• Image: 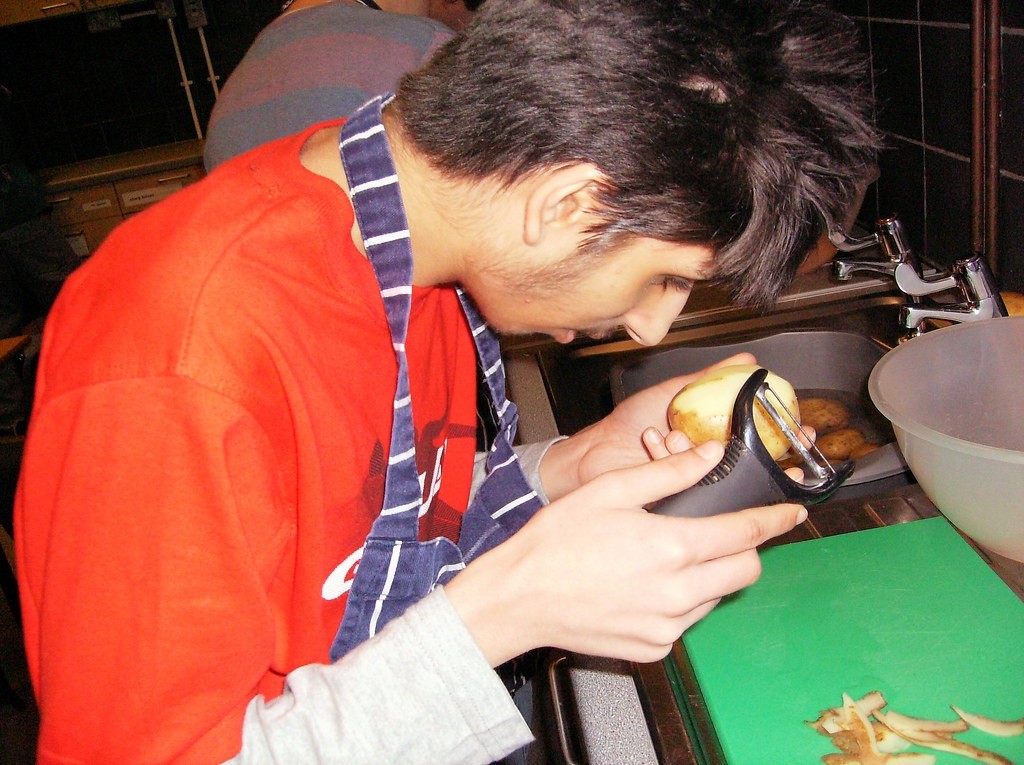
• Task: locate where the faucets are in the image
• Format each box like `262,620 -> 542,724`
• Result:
891,251 -> 1011,327
828,213 -> 927,345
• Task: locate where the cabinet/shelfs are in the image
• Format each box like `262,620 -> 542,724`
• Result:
47,163 -> 206,259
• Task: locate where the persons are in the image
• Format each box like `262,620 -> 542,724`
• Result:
201,0 -> 485,178
14,0 -> 875,765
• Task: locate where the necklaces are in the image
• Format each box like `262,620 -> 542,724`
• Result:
281,0 -> 368,11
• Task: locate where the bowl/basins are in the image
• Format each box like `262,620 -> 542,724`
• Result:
867,315 -> 1024,562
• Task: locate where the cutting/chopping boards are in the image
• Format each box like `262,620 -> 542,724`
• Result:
682,516 -> 1024,765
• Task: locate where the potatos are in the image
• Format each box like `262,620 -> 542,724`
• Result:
776,398 -> 886,471
667,365 -> 801,463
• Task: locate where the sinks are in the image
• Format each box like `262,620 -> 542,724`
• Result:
606,324 -> 896,469
